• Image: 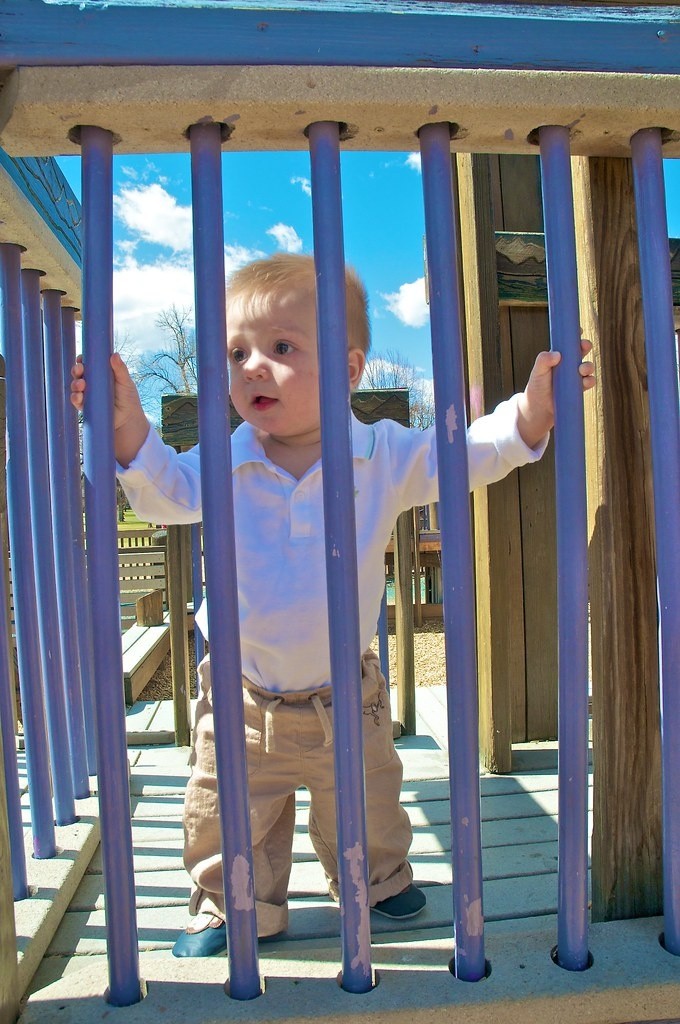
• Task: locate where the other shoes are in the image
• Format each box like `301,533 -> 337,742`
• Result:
171,913 -> 227,958
370,883 -> 427,919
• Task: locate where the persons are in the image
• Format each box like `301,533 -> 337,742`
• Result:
69,254 -> 598,962
